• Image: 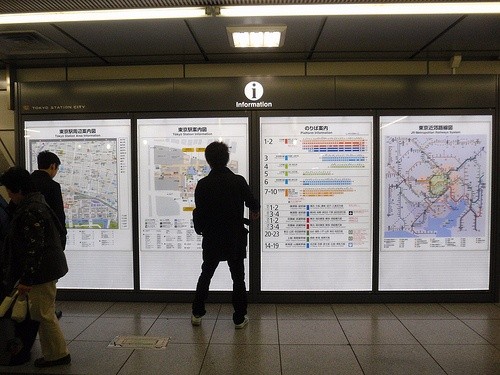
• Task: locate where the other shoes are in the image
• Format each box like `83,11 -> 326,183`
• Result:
33,354 -> 71,367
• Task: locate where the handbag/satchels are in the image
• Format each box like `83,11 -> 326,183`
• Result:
0,290 -> 40,366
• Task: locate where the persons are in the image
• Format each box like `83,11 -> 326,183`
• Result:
29,151 -> 68,318
192,141 -> 252,328
0,166 -> 71,367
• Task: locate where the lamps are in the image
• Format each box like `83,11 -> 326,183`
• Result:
0,6 -> 212,24
213,2 -> 500,17
226,26 -> 288,49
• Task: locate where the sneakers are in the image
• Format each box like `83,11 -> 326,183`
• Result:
235,316 -> 249,328
191,313 -> 202,325
